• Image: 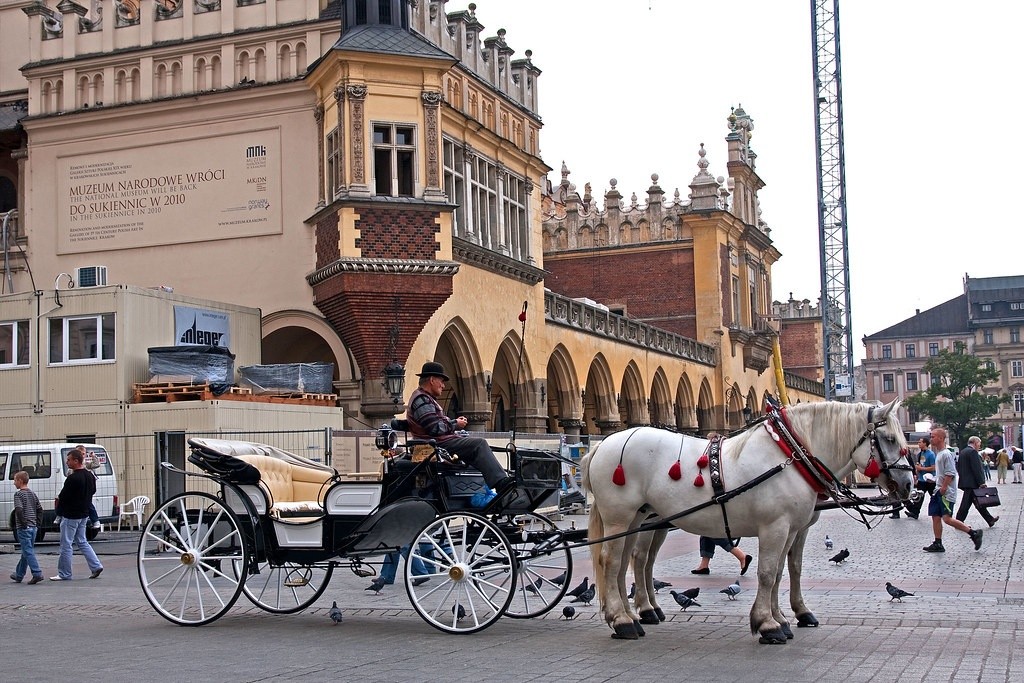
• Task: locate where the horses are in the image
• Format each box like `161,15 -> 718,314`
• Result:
577,395 -> 916,645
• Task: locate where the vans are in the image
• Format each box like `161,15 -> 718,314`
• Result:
0,442 -> 120,543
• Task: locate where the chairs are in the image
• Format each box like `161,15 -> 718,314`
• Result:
117,496 -> 151,531
391,418 -> 468,472
37,465 -> 51,477
22,466 -> 34,478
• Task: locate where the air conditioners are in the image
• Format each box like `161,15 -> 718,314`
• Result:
75,266 -> 108,289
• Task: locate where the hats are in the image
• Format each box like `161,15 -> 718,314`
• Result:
415,362 -> 450,382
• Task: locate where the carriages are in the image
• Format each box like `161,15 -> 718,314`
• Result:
135,394 -> 917,647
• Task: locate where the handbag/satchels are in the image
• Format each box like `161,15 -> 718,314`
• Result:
995,459 -> 1000,467
973,486 -> 1001,508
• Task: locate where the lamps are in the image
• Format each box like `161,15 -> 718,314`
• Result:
725,375 -> 752,425
379,298 -> 406,414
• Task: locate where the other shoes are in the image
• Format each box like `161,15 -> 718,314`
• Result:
969,529 -> 983,551
94,521 -> 101,529
412,577 -> 430,586
89,569 -> 103,580
372,578 -> 390,584
49,575 -> 62,581
904,511 -> 917,519
922,541 -> 945,552
740,554 -> 753,575
889,514 -> 900,519
989,516 -> 999,527
27,575 -> 43,585
496,476 -> 518,493
54,516 -> 62,524
440,553 -> 454,572
691,568 -> 710,575
10,574 -> 22,583
1018,481 -> 1022,483
1012,481 -> 1018,483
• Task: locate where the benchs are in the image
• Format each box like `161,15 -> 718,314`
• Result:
233,453 -> 332,523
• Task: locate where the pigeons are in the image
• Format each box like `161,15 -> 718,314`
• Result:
329,601 -> 344,625
842,548 -> 850,562
627,582 -> 636,599
452,599 -> 467,622
570,584 -> 596,606
719,579 -> 741,601
653,578 -> 672,594
519,575 -> 543,596
566,576 -> 590,597
828,548 -> 845,565
364,576 -> 386,596
548,570 -> 567,586
884,582 -> 915,603
824,534 -> 834,550
679,587 -> 701,601
563,606 -> 575,620
669,590 -> 702,612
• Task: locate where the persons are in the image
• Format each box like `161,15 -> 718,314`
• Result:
406,362 -> 515,492
889,436 -> 1024,520
49,445 -> 103,581
10,471 -> 44,585
371,443 -> 455,586
691,531 -> 753,577
923,428 -> 983,553
